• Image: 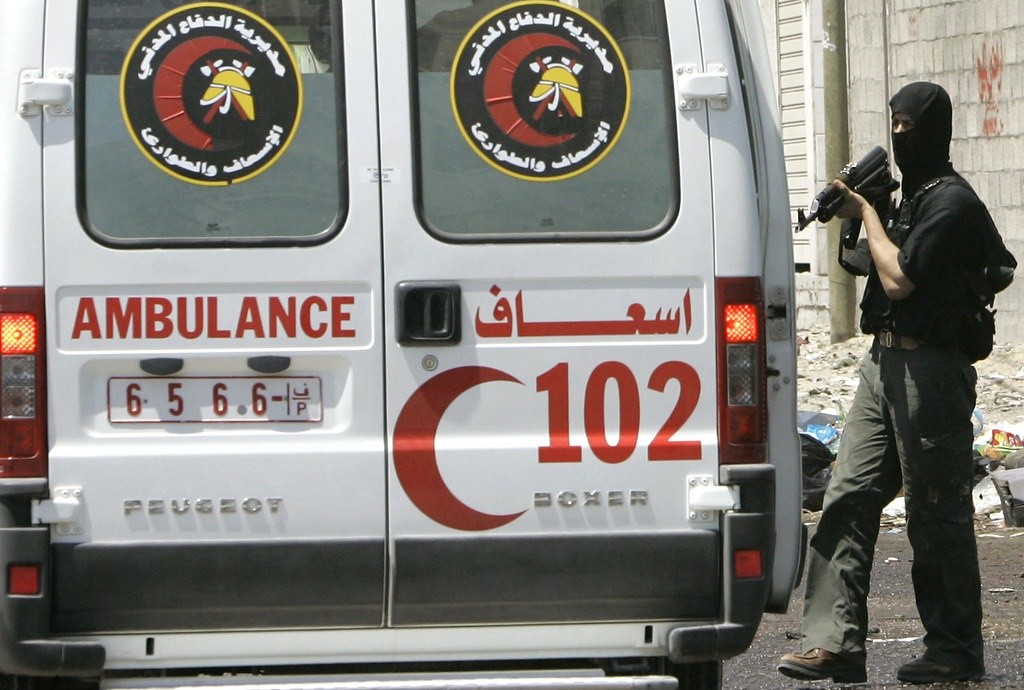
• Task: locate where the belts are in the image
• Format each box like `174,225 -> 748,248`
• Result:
876,328 -> 927,350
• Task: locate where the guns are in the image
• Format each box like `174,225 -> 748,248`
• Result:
790,143 -> 902,255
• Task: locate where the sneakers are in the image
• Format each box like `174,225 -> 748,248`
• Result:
898,656 -> 985,682
778,648 -> 867,683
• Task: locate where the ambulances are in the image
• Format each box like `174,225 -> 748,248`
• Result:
1,1 -> 810,690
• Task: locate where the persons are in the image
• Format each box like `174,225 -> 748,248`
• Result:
778,82 -> 1017,683
